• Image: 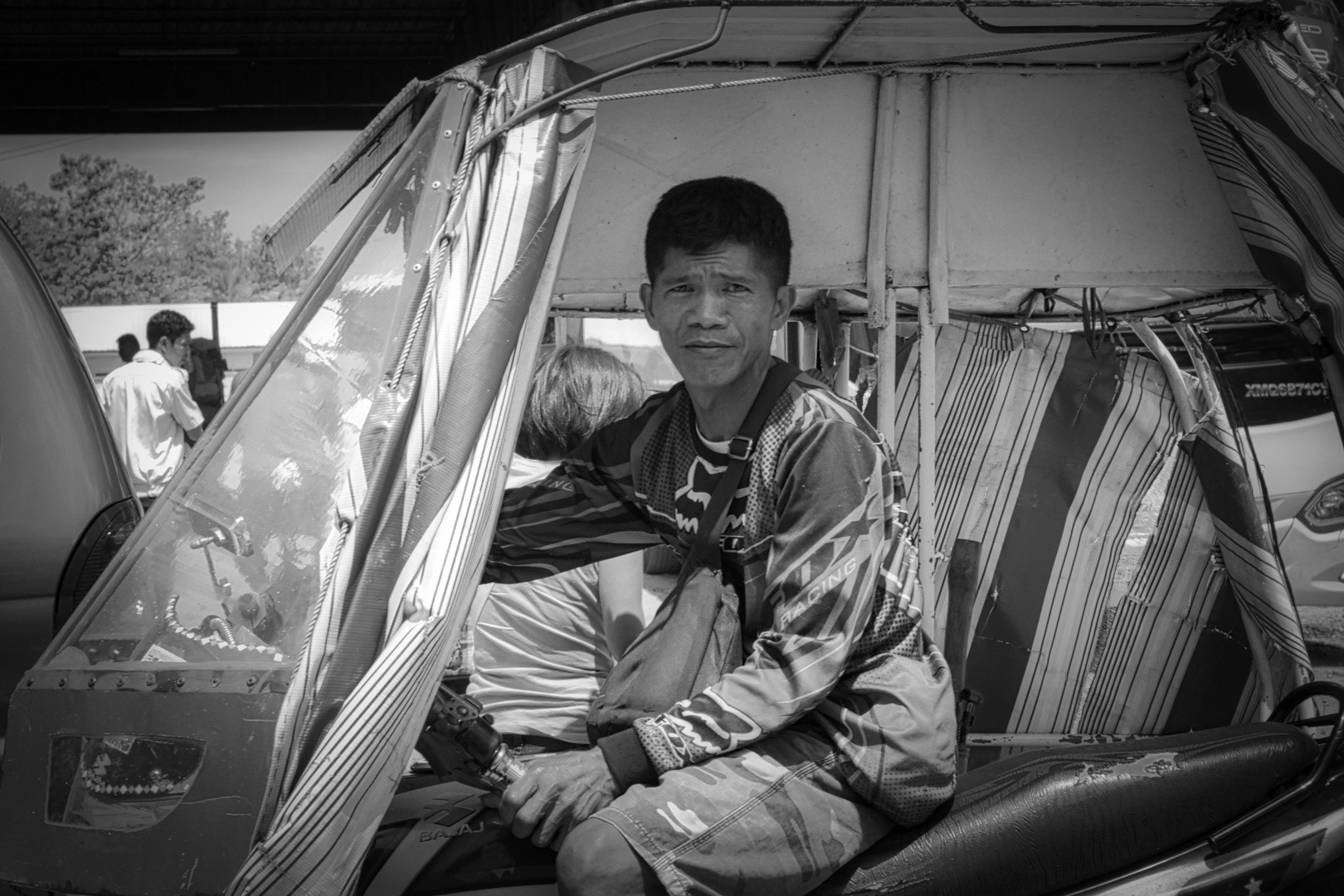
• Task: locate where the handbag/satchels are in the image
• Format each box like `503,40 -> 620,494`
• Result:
584,549 -> 746,748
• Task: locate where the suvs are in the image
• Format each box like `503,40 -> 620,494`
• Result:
1,218 -> 148,717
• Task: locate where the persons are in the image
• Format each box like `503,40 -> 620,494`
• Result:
460,346 -> 646,748
101,308 -> 227,615
231,335 -> 344,591
488,177 -> 957,896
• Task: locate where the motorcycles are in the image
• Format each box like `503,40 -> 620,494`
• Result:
0,0 -> 1344,896
